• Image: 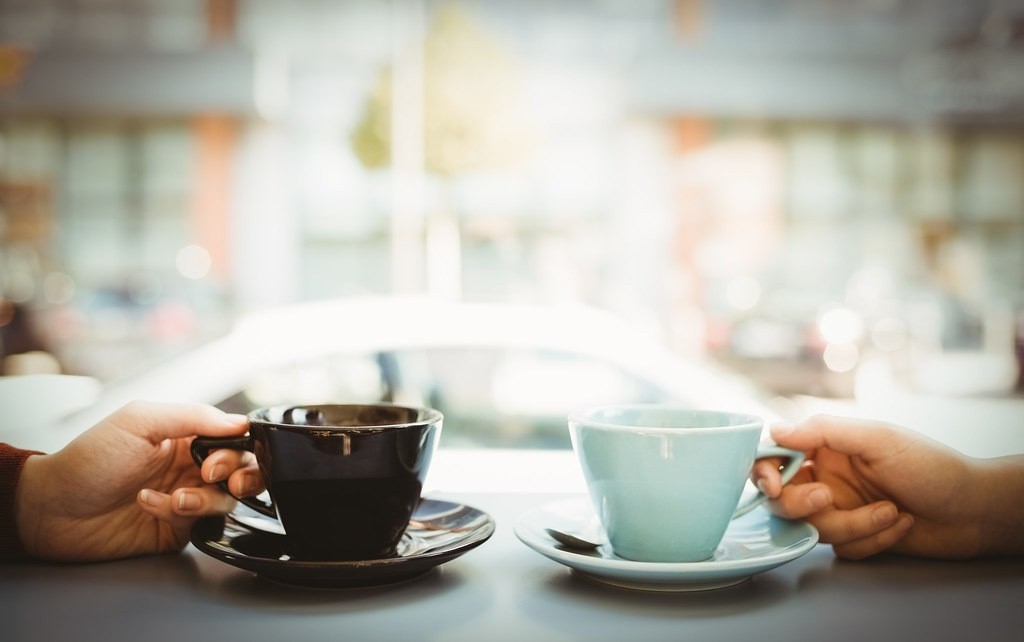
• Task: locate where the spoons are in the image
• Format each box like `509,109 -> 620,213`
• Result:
544,525 -> 605,551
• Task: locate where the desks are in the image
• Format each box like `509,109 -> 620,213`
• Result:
0,446 -> 1024,642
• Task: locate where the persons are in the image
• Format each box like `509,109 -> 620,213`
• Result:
749,413 -> 1024,562
0,398 -> 266,565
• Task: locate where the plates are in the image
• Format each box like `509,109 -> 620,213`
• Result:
513,486 -> 819,592
190,493 -> 495,588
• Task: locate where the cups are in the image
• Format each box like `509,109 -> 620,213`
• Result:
567,406 -> 805,562
190,402 -> 443,559
